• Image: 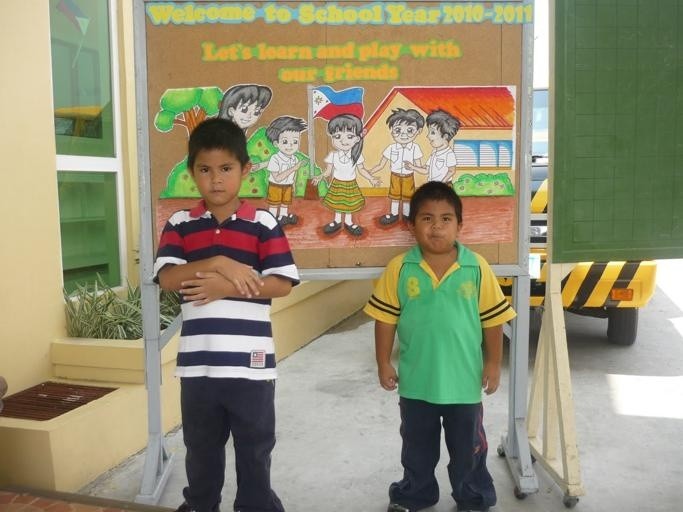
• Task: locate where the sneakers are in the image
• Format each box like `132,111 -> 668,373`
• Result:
275,213 -> 299,226
387,501 -> 415,512
378,214 -> 410,226
174,501 -> 244,512
323,220 -> 364,237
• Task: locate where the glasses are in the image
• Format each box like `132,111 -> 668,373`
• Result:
391,133 -> 415,138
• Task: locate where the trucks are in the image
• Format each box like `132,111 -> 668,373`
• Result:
490,1 -> 682,344
56,98 -> 104,139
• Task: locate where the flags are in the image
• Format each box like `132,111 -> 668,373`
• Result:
313,85 -> 364,124
56,0 -> 90,35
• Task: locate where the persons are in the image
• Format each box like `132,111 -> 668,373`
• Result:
153,118 -> 300,511
363,181 -> 518,512
312,114 -> 379,235
403,108 -> 460,190
217,84 -> 272,134
370,108 -> 424,225
265,115 -> 310,229
